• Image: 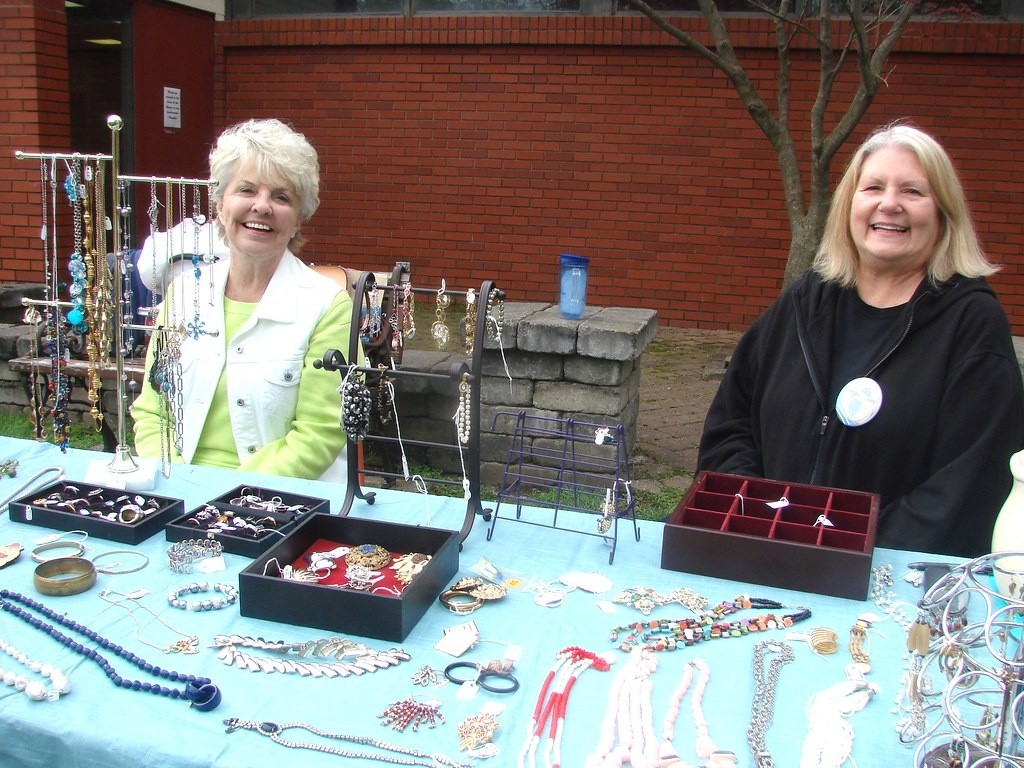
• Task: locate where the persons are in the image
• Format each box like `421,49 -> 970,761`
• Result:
696,126 -> 1024,561
128,119 -> 366,484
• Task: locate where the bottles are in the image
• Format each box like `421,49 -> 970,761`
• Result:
990,449 -> 1024,617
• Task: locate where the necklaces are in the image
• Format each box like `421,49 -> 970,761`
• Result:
209,634 -> 412,678
26,153 -> 213,482
523,592 -> 811,768
223,717 -> 475,768
0,589 -> 222,710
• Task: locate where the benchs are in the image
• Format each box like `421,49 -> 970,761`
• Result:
9,354 -> 148,452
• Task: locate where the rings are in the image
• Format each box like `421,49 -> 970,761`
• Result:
189,486 -> 310,537
34,485 -> 159,523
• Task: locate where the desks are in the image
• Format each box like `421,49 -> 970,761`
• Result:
0,434 -> 1024,768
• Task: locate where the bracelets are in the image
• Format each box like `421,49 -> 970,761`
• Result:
32,541 -> 97,596
339,365 -> 471,445
91,549 -> 148,574
372,281 -> 504,351
167,582 -> 238,612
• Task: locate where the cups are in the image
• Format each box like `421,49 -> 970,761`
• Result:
558,253 -> 590,320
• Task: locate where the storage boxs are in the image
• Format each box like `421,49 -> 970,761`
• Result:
662,470 -> 882,601
237,514 -> 459,645
166,484 -> 331,561
10,478 -> 184,547
